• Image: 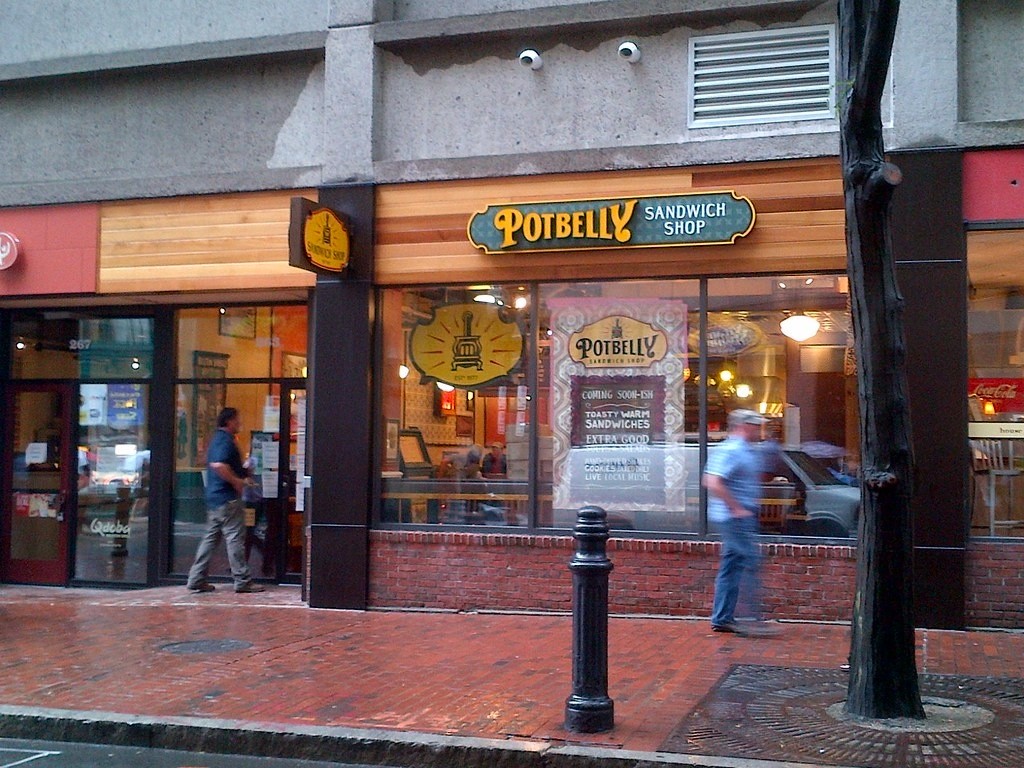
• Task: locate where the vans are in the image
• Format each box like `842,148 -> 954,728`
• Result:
552,442 -> 862,538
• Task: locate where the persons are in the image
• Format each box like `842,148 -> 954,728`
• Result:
700,408 -> 782,636
437,442 -> 507,476
187,407 -> 284,592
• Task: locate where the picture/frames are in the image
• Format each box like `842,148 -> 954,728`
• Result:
220,306 -> 257,340
386,417 -> 400,463
456,415 -> 473,437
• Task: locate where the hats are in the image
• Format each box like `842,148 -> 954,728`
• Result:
729,409 -> 773,425
492,442 -> 504,449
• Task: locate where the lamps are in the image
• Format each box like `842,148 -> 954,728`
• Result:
780,310 -> 820,341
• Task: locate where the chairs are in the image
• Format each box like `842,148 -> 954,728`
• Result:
967,394 -> 1024,537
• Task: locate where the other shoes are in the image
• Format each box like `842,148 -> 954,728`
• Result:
711,618 -> 747,633
737,623 -> 783,637
237,582 -> 265,592
188,582 -> 215,591
